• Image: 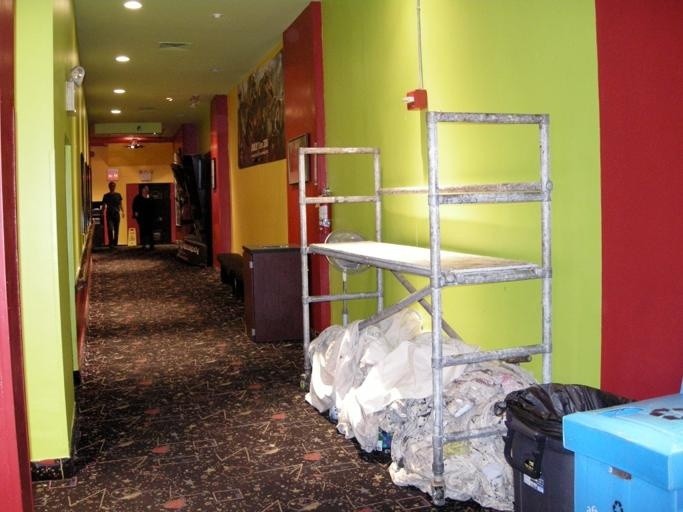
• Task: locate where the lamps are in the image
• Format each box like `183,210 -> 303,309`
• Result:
65,66 -> 86,112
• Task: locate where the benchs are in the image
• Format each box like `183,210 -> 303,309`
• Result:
217,253 -> 244,298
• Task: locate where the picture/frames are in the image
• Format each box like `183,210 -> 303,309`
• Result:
287,133 -> 310,185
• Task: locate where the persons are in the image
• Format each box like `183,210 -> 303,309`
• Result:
102,181 -> 124,246
132,185 -> 154,250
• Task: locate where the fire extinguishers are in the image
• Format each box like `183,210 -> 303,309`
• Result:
317,190 -> 331,242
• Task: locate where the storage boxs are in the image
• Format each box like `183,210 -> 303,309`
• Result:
561,390 -> 682,511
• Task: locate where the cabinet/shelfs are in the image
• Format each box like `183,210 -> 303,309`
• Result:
242,244 -> 311,344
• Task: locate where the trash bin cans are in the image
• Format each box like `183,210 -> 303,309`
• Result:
504,383 -> 634,512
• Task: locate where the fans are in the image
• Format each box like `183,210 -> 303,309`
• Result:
324,229 -> 371,332
